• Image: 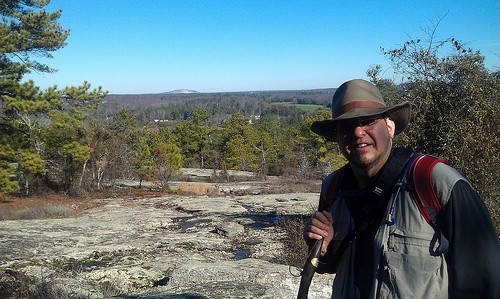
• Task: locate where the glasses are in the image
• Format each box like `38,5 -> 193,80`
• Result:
325,116 -> 388,141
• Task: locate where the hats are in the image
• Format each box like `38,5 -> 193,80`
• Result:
310,79 -> 411,142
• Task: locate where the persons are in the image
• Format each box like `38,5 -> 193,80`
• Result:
303,79 -> 500,299
